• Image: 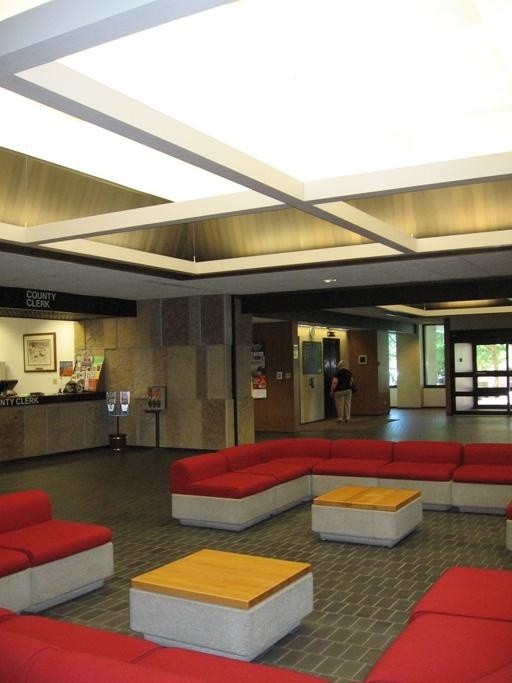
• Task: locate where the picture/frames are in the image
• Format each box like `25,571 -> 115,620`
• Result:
23,333 -> 56,372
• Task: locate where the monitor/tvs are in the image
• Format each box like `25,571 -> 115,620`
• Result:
0,379 -> 19,398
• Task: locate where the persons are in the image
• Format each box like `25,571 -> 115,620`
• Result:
331,359 -> 354,423
253,369 -> 267,389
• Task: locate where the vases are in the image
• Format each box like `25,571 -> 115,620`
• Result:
109,433 -> 126,458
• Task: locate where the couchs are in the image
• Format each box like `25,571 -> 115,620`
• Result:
170,438 -> 512,552
0,489 -> 512,683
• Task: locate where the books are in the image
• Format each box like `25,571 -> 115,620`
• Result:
71,356 -> 102,392
30,392 -> 45,396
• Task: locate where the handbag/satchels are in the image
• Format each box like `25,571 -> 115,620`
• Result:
351,383 -> 357,393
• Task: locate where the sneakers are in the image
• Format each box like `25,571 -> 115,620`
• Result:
336,420 -> 342,423
344,420 -> 350,423
